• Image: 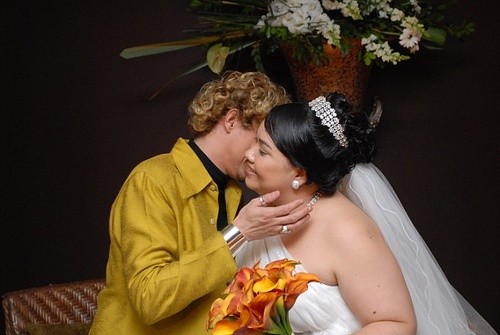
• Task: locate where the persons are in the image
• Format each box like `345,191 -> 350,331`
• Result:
236,90 -> 499,335
87,70 -> 313,335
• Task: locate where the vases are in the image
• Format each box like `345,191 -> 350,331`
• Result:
281,38 -> 371,113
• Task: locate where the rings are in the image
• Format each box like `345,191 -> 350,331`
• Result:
259,196 -> 267,206
280,225 -> 291,234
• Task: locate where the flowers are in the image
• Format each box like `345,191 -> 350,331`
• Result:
206,258 -> 322,335
120,0 -> 475,101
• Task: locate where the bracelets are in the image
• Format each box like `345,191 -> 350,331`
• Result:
221,223 -> 249,259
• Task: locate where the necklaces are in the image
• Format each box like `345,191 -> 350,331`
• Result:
308,190 -> 322,206
